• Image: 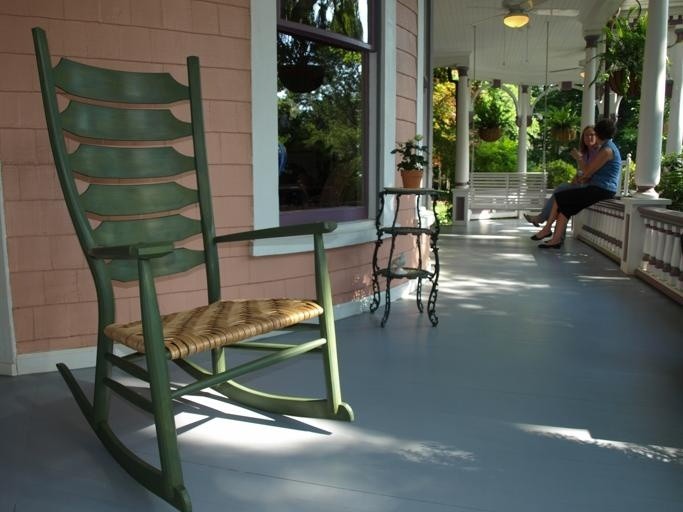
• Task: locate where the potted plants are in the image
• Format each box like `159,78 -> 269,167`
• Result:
586,1 -> 649,99
391,134 -> 432,188
540,100 -> 580,145
471,87 -> 512,142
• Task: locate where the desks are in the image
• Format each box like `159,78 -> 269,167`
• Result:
369,188 -> 441,326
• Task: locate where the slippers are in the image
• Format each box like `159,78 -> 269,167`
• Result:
524,213 -> 540,227
543,237 -> 564,244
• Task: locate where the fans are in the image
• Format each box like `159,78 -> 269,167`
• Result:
466,0 -> 579,30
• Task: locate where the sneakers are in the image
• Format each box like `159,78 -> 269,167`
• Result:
530,230 -> 552,240
538,244 -> 561,249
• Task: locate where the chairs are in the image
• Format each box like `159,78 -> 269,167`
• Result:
32,26 -> 354,511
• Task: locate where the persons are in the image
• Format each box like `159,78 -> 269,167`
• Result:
530,118 -> 623,249
523,123 -> 603,244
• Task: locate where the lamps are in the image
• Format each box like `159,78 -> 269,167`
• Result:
502,14 -> 529,30
